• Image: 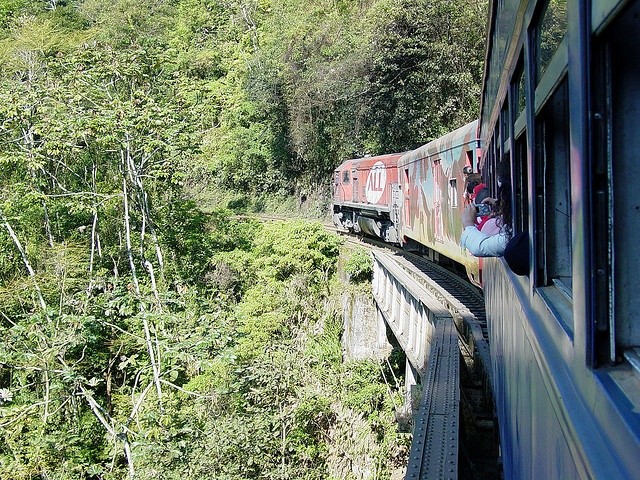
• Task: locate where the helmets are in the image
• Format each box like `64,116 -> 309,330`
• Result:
463,166 -> 472,174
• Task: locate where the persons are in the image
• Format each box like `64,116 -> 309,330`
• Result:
460,165 -> 511,257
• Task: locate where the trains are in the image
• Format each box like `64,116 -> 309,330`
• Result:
334,0 -> 639,480
